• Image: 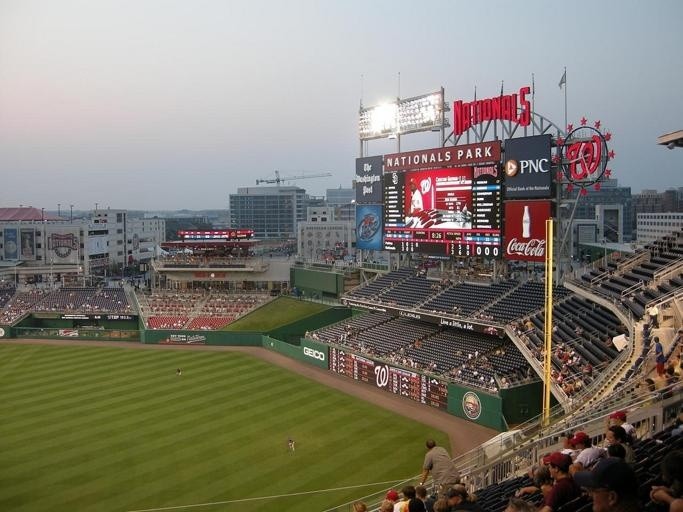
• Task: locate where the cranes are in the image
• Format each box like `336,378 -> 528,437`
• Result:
256,171 -> 332,186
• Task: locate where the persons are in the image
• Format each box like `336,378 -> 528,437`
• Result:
0,289 -> 132,323
347,410 -> 683,512
406,176 -> 424,212
509,318 -> 593,401
648,303 -> 659,327
638,337 -> 683,398
393,278 -> 496,322
306,325 -> 505,389
145,319 -> 212,330
289,439 -> 295,451
176,367 -> 181,375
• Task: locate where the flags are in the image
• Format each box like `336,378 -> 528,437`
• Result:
559,70 -> 568,88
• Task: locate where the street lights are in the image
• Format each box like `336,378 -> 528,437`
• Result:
359,85 -> 443,156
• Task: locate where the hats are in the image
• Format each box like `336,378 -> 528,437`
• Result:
544,452 -> 566,466
574,457 -> 636,491
609,411 -> 627,421
384,490 -> 398,502
570,432 -> 587,445
